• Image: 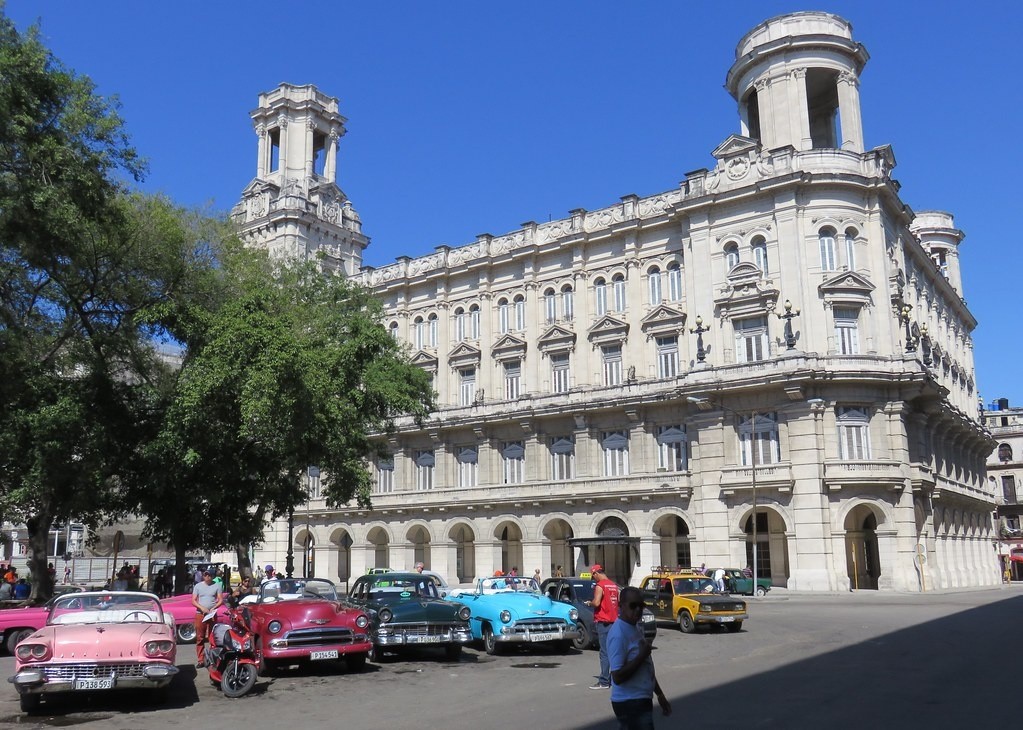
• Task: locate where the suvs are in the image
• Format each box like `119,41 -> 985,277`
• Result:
637,565 -> 749,634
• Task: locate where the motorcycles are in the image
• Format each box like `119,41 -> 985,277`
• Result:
201,595 -> 262,698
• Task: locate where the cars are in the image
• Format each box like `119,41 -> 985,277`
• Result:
237,577 -> 372,676
343,568 -> 473,663
0,583 -> 232,713
702,567 -> 772,597
442,576 -> 658,655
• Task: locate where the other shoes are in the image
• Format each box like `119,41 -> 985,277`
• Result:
196,663 -> 204,668
589,683 -> 610,690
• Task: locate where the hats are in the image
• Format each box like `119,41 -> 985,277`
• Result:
202,570 -> 210,575
589,565 -> 604,581
264,566 -> 273,572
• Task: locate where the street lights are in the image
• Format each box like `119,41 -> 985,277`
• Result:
686,395 -> 824,597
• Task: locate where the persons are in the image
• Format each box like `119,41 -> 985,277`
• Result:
606,587 -> 671,730
104,554 -> 289,604
742,564 -> 753,578
699,562 -> 707,576
46,561 -> 56,575
191,569 -> 223,668
533,568 -> 541,585
60,562 -> 73,585
657,563 -> 682,588
404,561 -> 424,595
715,567 -> 725,592
0,563 -> 31,602
505,565 -> 518,591
584,564 -> 620,691
493,570 -> 507,589
554,565 -> 566,577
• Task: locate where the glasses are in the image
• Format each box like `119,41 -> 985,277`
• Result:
624,602 -> 647,610
244,580 -> 249,582
